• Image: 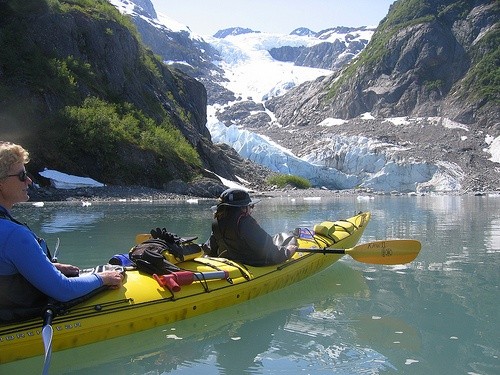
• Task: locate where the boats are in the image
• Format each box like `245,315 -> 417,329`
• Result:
0,212 -> 375,363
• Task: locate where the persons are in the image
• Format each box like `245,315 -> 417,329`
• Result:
0,142 -> 124,324
211,187 -> 299,267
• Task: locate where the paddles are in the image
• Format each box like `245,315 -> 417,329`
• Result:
36,237 -> 60,375
295,239 -> 422,266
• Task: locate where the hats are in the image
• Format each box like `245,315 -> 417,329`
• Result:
218,188 -> 264,207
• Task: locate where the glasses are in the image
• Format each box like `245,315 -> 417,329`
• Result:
1,168 -> 27,182
246,203 -> 255,209
